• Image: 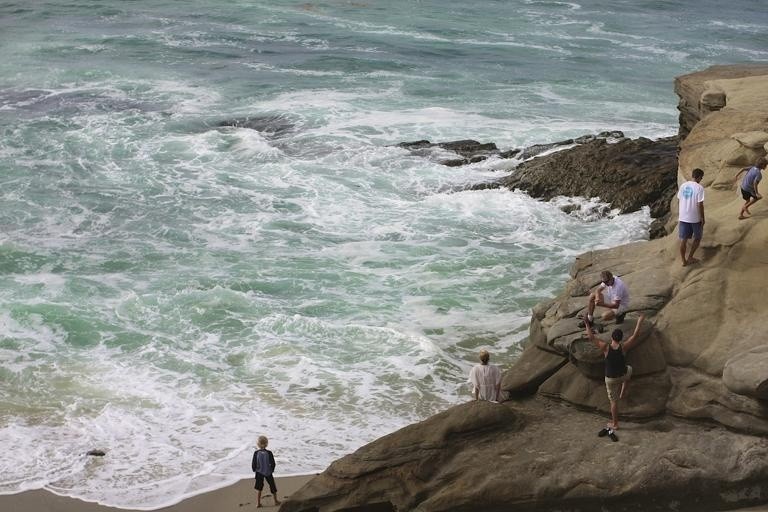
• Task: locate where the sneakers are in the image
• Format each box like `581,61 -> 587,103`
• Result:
577,318 -> 594,328
598,427 -> 618,442
615,312 -> 626,324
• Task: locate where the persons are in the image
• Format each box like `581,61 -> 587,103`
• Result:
677,168 -> 706,266
471,349 -> 510,403
578,270 -> 629,327
252,435 -> 282,507
737,156 -> 767,219
583,312 -> 645,430
